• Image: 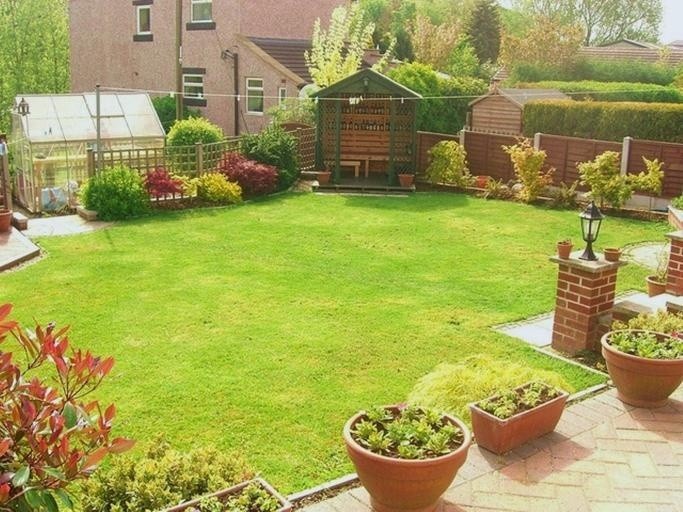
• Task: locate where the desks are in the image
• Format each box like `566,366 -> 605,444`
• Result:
323,152 -> 412,178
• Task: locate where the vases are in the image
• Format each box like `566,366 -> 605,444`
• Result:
604,247 -> 621,262
398,173 -> 415,186
315,171 -> 332,185
0,208 -> 12,233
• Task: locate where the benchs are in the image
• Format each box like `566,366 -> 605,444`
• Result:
323,160 -> 361,177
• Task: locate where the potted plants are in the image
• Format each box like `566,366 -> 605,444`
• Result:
163,476 -> 293,512
343,380 -> 571,511
557,239 -> 573,259
600,329 -> 683,407
645,237 -> 672,298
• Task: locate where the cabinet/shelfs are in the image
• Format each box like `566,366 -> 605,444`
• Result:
321,102 -> 413,135
